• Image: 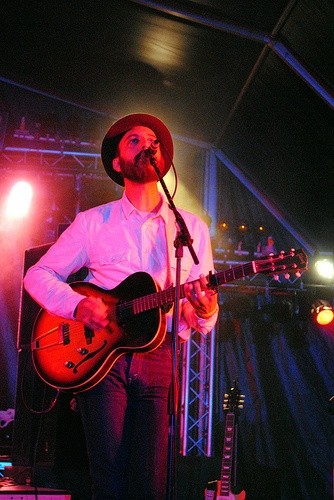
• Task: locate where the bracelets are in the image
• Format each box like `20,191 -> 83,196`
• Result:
196,306 -> 218,318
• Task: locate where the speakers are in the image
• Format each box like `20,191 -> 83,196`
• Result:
9,243 -> 89,492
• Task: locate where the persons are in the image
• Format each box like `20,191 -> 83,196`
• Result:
262,234 -> 279,254
23,112 -> 222,499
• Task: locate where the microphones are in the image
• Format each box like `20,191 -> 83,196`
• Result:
144,137 -> 160,158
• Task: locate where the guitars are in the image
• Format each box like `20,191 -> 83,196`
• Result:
29,244 -> 308,394
204,376 -> 246,500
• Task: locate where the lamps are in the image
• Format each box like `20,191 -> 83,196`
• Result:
308,299 -> 333,325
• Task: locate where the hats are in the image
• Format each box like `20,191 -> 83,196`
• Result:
100,113 -> 174,187
